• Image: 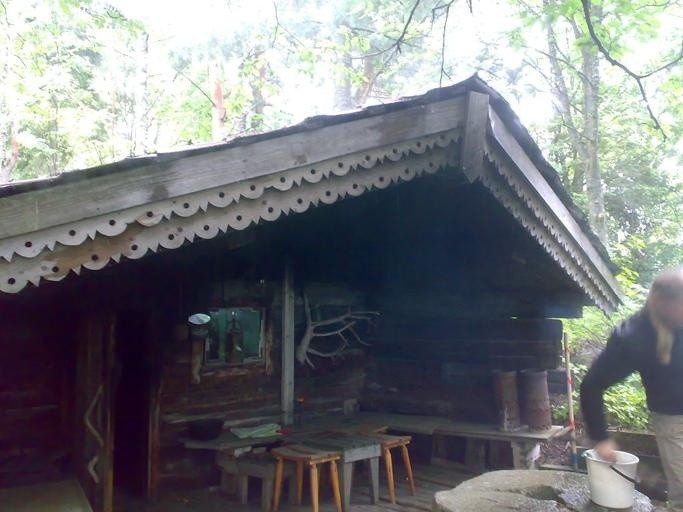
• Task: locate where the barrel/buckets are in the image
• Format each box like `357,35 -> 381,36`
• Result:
489,365 -> 519,430
583,450 -> 641,509
520,367 -> 551,433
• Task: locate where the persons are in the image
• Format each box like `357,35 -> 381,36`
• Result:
579,270 -> 683,512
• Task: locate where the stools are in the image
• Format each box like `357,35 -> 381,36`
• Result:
183,424 -> 414,510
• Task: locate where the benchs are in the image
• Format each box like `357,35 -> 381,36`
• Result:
359,412 -> 564,470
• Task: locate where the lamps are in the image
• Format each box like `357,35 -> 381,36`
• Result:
185,293 -> 212,326
